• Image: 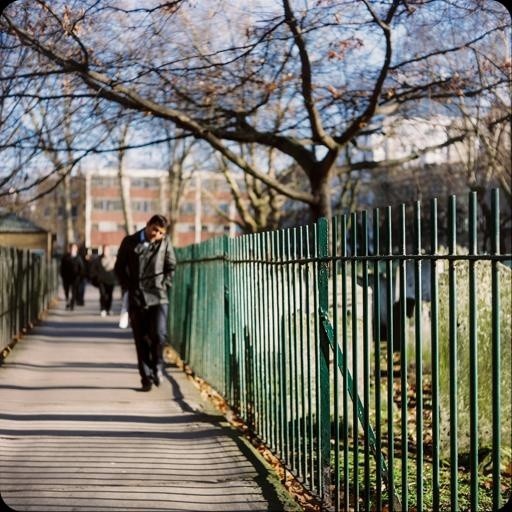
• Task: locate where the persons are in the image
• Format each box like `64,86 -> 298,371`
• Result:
118,290 -> 130,329
75,245 -> 93,307
91,244 -> 117,315
59,243 -> 85,309
114,214 -> 179,390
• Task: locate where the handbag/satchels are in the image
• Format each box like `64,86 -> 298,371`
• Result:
119,291 -> 129,328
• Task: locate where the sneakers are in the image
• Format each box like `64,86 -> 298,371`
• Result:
101,309 -> 114,317
143,384 -> 152,391
154,372 -> 161,388
